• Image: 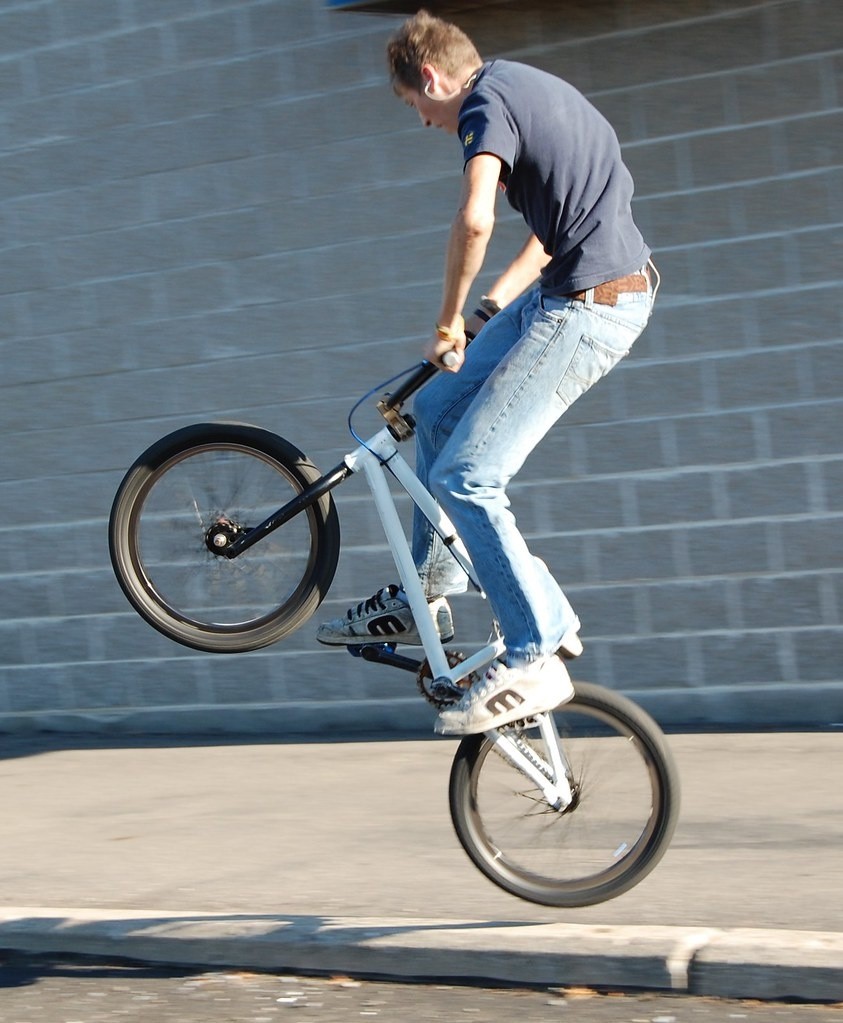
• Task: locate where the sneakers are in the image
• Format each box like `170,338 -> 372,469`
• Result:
434,654 -> 575,736
316,583 -> 454,646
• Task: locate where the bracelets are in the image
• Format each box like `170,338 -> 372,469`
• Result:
436,313 -> 464,341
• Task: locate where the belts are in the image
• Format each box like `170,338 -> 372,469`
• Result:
561,266 -> 650,306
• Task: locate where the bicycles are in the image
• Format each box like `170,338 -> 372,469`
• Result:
106,328 -> 684,910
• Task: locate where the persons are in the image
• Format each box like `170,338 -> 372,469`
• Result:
314,11 -> 662,732
